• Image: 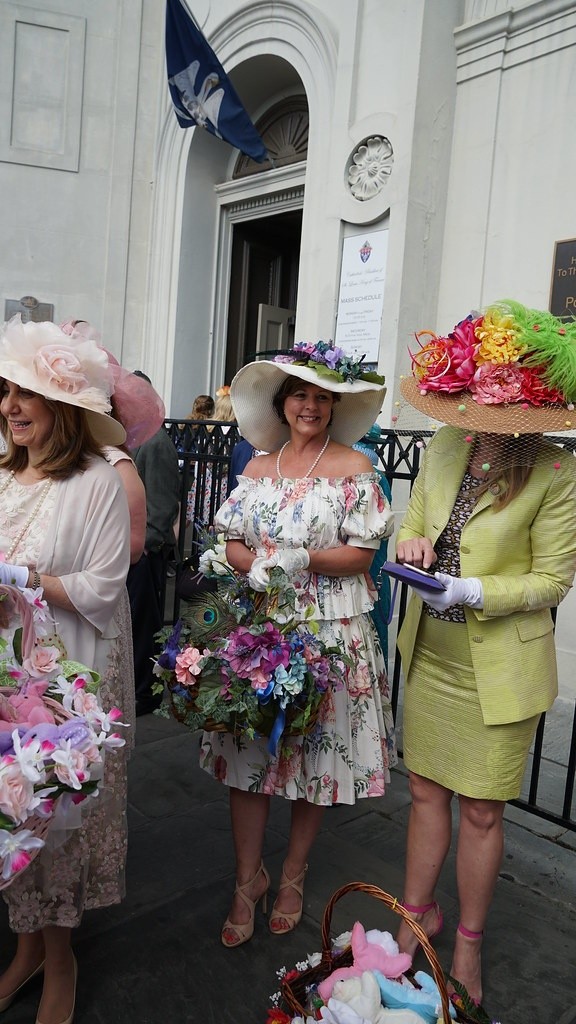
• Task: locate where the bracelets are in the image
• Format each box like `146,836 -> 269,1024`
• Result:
33,572 -> 40,591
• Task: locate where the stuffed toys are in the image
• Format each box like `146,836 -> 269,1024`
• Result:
289,920 -> 457,1024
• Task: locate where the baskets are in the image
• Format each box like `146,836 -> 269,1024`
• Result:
170,591 -> 329,744
282,882 -> 481,1024
0,584 -> 80,892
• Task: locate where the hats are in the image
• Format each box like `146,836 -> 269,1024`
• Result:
60,316 -> 165,447
229,340 -> 388,454
0,312 -> 126,447
397,300 -> 576,469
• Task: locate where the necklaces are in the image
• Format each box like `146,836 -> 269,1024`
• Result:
0,468 -> 53,564
277,434 -> 330,478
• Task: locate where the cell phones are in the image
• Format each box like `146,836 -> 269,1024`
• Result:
403,562 -> 436,579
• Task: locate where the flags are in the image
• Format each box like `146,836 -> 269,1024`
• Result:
165,0 -> 267,165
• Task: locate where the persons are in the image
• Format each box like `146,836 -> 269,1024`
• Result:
397,298 -> 576,1011
186,385 -> 242,524
130,370 -> 179,716
232,440 -> 270,492
214,340 -> 397,948
351,423 -> 390,674
0,313 -> 130,1024
165,395 -> 215,576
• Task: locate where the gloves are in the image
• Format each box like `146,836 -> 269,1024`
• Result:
261,546 -> 310,580
248,558 -> 271,592
411,571 -> 483,613
0,561 -> 28,588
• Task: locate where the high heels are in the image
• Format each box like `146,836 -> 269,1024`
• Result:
446,923 -> 484,1008
0,954 -> 47,1010
396,899 -> 442,965
269,862 -> 309,934
36,946 -> 77,1024
222,859 -> 270,947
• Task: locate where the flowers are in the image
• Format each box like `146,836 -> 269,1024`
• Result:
0,312 -> 116,413
269,338 -> 386,385
0,577 -> 132,882
216,386 -> 231,398
407,307 -> 567,407
265,929 -> 353,1024
149,515 -> 355,759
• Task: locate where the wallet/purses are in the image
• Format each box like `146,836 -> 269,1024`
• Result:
381,562 -> 447,594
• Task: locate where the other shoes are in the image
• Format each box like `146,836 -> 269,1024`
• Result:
166,566 -> 176,578
